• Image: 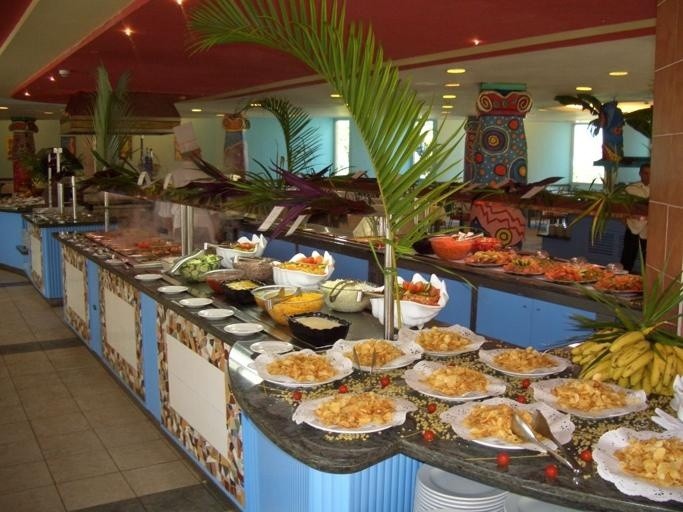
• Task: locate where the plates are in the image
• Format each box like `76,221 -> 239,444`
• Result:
334,338 -> 425,371
133,273 -> 162,282
157,286 -> 190,294
403,359 -> 506,400
292,391 -> 419,435
253,347 -> 354,386
397,326 -> 485,356
598,429 -> 683,501
534,379 -> 644,418
477,345 -> 573,378
464,230 -> 643,298
58,228 -> 125,265
446,400 -> 567,452
408,462 -> 510,511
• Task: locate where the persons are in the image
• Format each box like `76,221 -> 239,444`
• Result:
157,143 -> 217,241
616,162 -> 651,272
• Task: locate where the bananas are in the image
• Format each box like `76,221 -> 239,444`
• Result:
571,329 -> 682,395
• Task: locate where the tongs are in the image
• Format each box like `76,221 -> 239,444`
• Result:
505,407 -> 584,476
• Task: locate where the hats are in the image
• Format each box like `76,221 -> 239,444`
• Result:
169,120 -> 201,155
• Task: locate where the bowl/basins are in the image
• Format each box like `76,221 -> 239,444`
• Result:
410,235 -> 474,260
174,242 -> 442,348
